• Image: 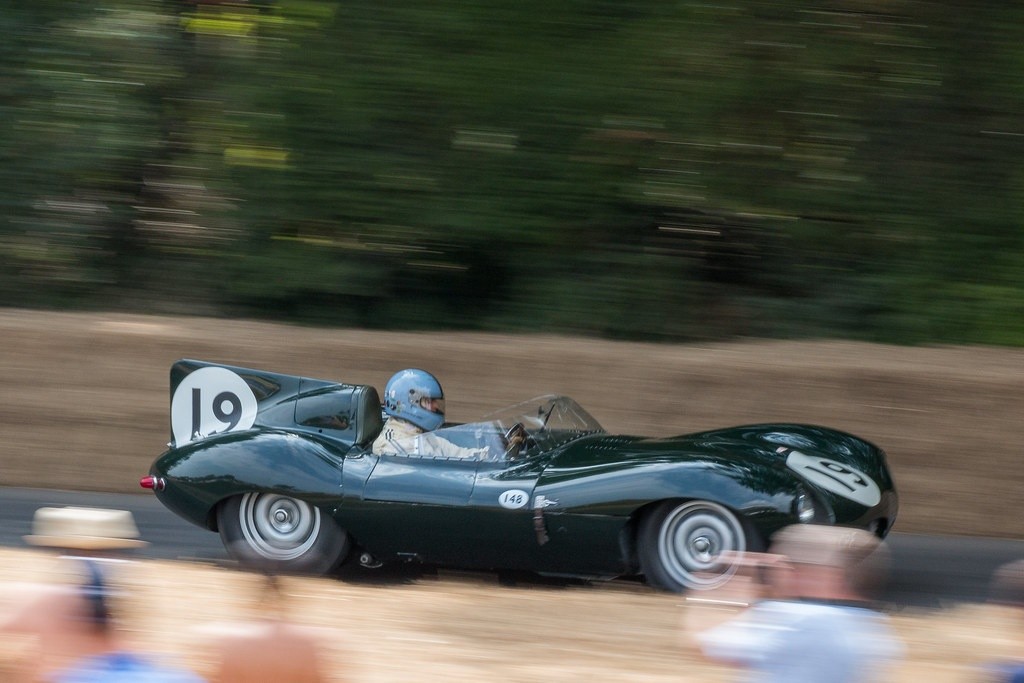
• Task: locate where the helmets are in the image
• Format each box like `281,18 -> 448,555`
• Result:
384,368 -> 446,431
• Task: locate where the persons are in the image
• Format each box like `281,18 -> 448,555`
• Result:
0,522 -> 1024,683
373,369 -> 523,461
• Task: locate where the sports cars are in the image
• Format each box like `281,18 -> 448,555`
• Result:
137,358 -> 901,601
